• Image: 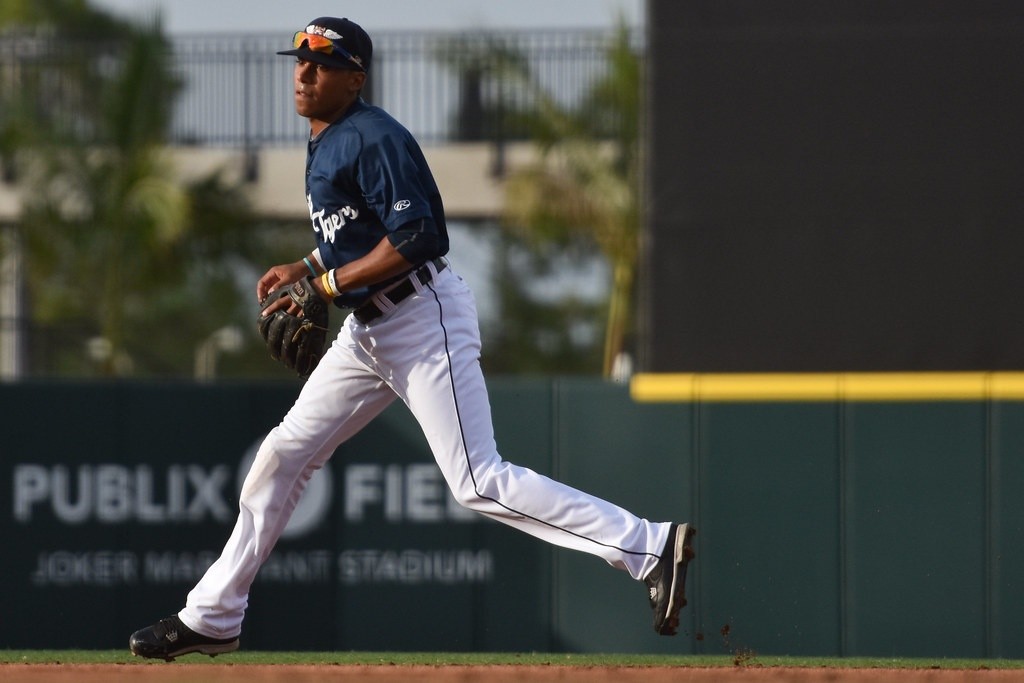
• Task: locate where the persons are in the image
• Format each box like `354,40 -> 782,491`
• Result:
129,17 -> 697,660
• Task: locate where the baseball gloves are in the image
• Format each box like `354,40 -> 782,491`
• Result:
257,275 -> 329,379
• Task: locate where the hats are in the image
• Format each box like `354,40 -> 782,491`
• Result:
276,17 -> 373,74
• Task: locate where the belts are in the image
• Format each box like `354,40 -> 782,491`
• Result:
351,257 -> 447,325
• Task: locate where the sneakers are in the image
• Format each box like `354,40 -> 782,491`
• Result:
129,614 -> 240,662
644,522 -> 696,635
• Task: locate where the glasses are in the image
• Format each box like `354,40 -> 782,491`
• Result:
292,30 -> 367,74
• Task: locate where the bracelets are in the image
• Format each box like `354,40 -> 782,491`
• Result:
303,257 -> 318,278
322,268 -> 342,298
